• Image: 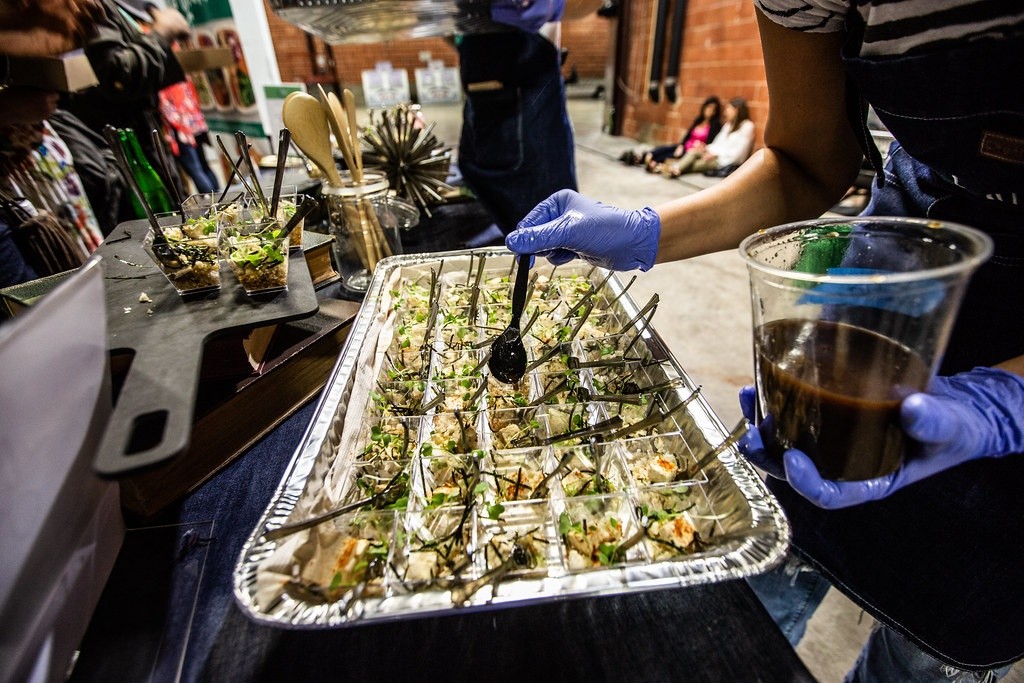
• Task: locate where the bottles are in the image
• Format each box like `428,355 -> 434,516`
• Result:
319,167 -> 404,294
116,127 -> 174,218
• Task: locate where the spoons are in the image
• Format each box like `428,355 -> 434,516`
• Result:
115,153 -> 182,270
487,253 -> 530,384
266,484 -> 408,539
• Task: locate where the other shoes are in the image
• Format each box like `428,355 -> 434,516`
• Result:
644,153 -> 656,172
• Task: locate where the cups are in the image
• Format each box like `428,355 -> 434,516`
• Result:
742,214 -> 996,480
286,263 -> 724,602
244,186 -> 304,251
180,191 -> 243,239
143,215 -> 222,298
221,214 -> 289,297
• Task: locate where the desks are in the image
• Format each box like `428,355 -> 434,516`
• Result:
68,154 -> 822,683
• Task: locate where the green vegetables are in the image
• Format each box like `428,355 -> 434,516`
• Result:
330,269 -> 692,586
165,188 -> 304,269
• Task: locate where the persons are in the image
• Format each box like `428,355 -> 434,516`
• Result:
621,96 -> 722,167
645,98 -> 755,177
0,0 -> 220,286
506,0 -> 1024,683
427,0 -> 564,245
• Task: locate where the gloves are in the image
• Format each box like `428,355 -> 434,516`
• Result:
739,367 -> 1024,511
505,189 -> 660,273
491,0 -> 565,33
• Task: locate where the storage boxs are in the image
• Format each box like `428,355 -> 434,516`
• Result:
0,255 -> 125,683
